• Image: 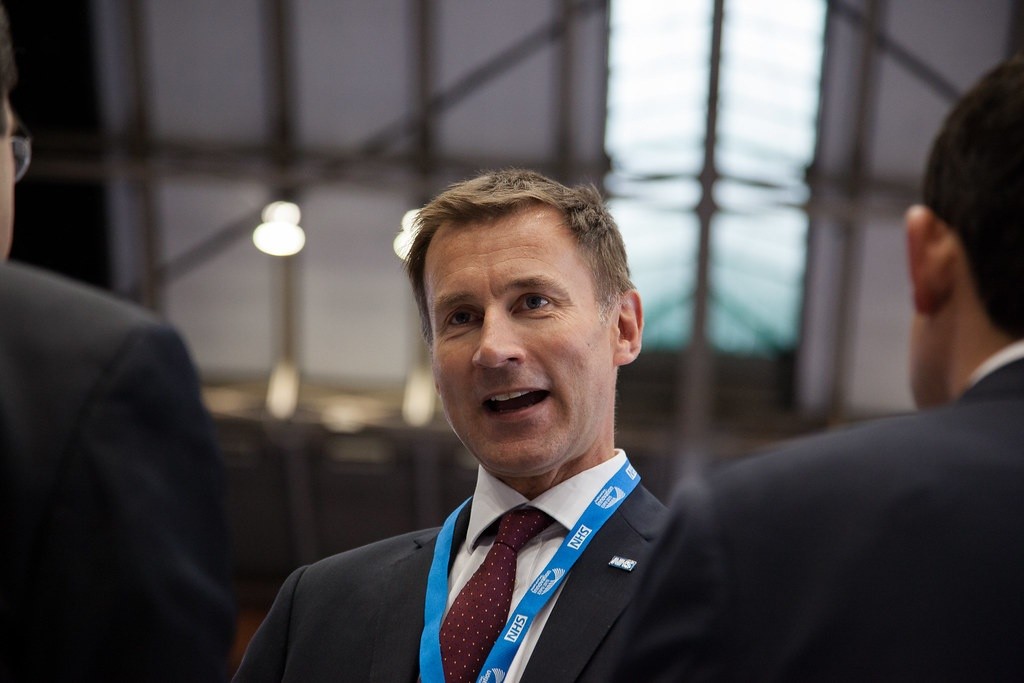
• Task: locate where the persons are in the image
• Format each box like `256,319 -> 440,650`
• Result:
235,169 -> 667,683
0,0 -> 236,683
615,52 -> 1024,683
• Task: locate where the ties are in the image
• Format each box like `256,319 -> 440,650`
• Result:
418,508 -> 557,682
2,136 -> 31,182
